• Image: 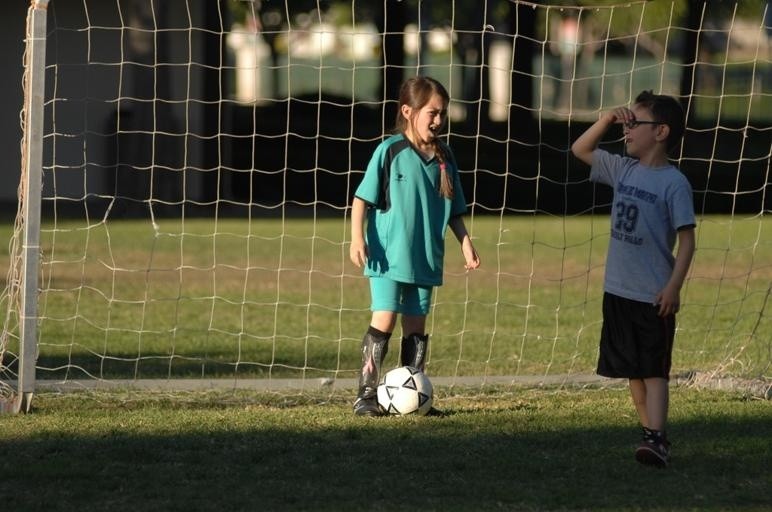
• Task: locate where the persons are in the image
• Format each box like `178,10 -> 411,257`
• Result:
570,93 -> 696,465
349,78 -> 482,414
224,3 -> 772,105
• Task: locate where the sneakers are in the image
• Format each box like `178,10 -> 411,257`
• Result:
353,386 -> 381,416
634,427 -> 672,468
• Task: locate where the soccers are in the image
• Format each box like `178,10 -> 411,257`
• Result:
376,365 -> 434,417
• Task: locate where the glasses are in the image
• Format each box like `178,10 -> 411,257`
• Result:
628,118 -> 664,128
